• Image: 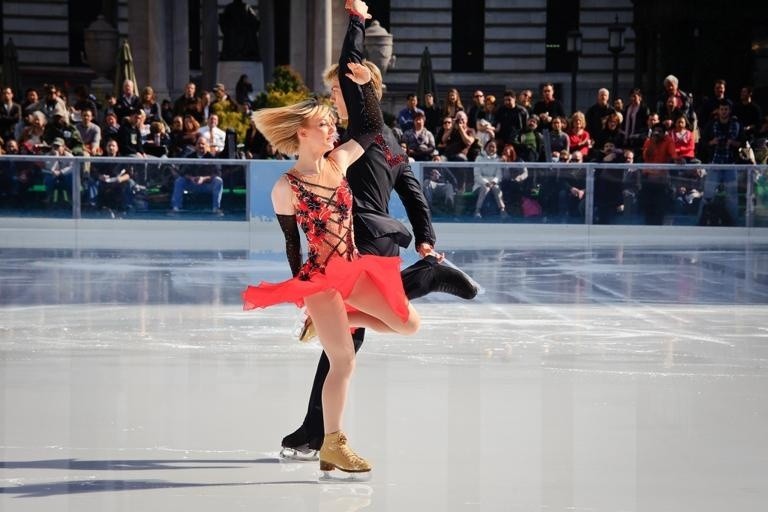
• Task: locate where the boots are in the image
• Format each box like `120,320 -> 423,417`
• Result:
298,314 -> 318,341
319,431 -> 372,474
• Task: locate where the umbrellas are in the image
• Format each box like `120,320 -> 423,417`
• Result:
415,46 -> 440,108
113,38 -> 139,98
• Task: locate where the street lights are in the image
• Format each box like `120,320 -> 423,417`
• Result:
565,22 -> 585,117
606,13 -> 627,111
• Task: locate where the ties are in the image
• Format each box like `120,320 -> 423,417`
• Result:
209,128 -> 214,145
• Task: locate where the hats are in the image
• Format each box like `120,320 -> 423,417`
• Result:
52,110 -> 65,117
52,137 -> 66,146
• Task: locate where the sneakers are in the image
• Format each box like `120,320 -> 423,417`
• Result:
281,423 -> 324,452
165,209 -> 225,218
424,254 -> 478,298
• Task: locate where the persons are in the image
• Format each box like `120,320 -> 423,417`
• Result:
281,0 -> 477,451
242,61 -> 419,473
1,73 -> 765,224
